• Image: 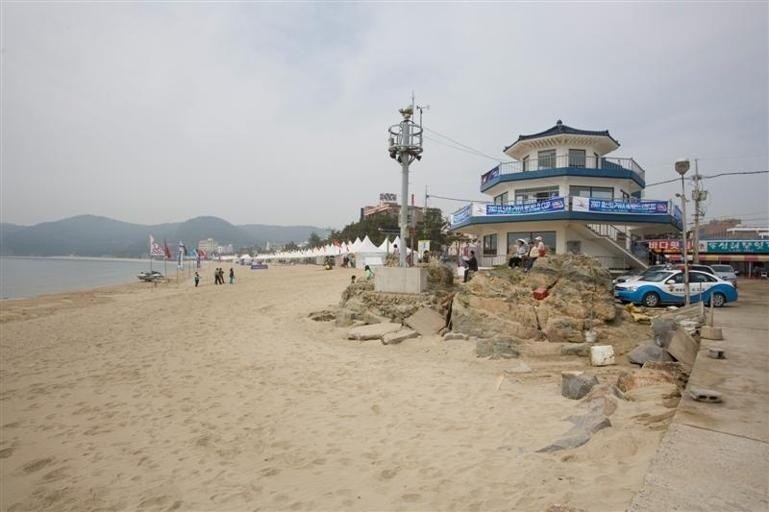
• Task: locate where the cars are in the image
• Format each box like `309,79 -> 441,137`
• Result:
611,260 -> 739,308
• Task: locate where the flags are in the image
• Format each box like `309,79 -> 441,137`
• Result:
147,235 -> 208,271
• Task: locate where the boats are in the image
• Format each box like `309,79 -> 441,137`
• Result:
136,270 -> 164,281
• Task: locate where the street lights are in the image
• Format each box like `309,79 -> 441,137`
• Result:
674,157 -> 689,310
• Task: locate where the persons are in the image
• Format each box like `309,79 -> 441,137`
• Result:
323,253 -> 356,270
364,265 -> 375,281
351,275 -> 356,284
392,244 -> 399,257
214,268 -> 224,284
229,268 -> 235,284
193,272 -> 199,287
508,236 -> 545,270
422,250 -> 430,262
462,250 -> 478,283
